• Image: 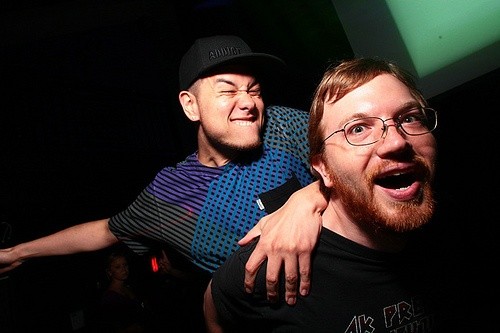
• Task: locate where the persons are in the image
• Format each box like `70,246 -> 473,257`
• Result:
94,249 -> 151,332
199,57 -> 500,333
1,39 -> 333,309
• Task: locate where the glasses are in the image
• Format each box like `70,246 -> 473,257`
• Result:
315,107 -> 438,152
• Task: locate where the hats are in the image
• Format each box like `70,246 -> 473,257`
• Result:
178,36 -> 288,95
